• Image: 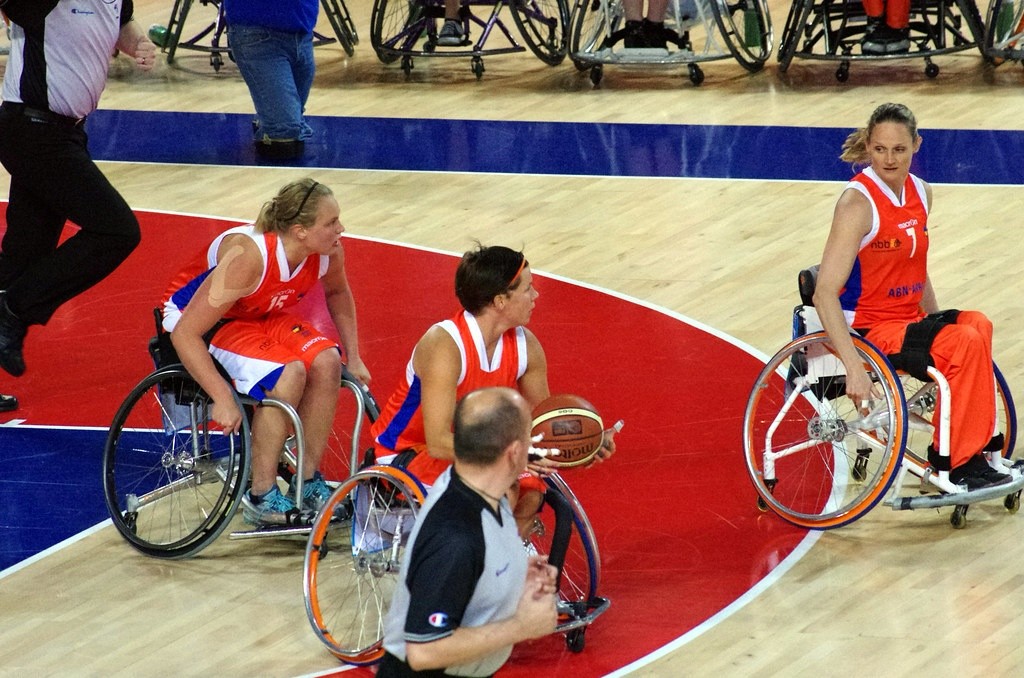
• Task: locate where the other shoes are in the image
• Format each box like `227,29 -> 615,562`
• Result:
557,595 -> 608,626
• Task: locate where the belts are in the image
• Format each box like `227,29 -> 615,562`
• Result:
1,101 -> 86,130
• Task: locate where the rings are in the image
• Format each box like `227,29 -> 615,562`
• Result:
142,58 -> 145,64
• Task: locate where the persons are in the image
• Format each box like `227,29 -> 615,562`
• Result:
371,246 -> 625,558
860,0 -> 911,55
813,103 -> 1013,494
624,0 -> 669,48
222,0 -> 320,144
162,176 -> 370,530
0,0 -> 158,411
376,386 -> 558,678
436,0 -> 463,45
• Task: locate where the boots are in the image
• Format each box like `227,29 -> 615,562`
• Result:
862,15 -> 886,54
624,18 -> 669,56
884,24 -> 911,54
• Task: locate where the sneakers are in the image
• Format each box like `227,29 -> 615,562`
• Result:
284,471 -> 350,521
958,467 -> 1013,492
437,19 -> 463,45
243,484 -> 312,527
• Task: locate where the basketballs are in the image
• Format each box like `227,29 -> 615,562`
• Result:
530,393 -> 605,469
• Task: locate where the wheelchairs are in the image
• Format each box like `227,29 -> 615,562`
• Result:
982,0 -> 1024,86
102,308 -> 382,561
370,0 -> 570,79
300,469 -> 611,666
777,0 -> 986,86
741,265 -> 1024,530
566,0 -> 773,88
149,0 -> 359,74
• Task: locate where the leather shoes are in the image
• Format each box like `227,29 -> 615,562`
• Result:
0,394 -> 18,412
0,291 -> 25,376
